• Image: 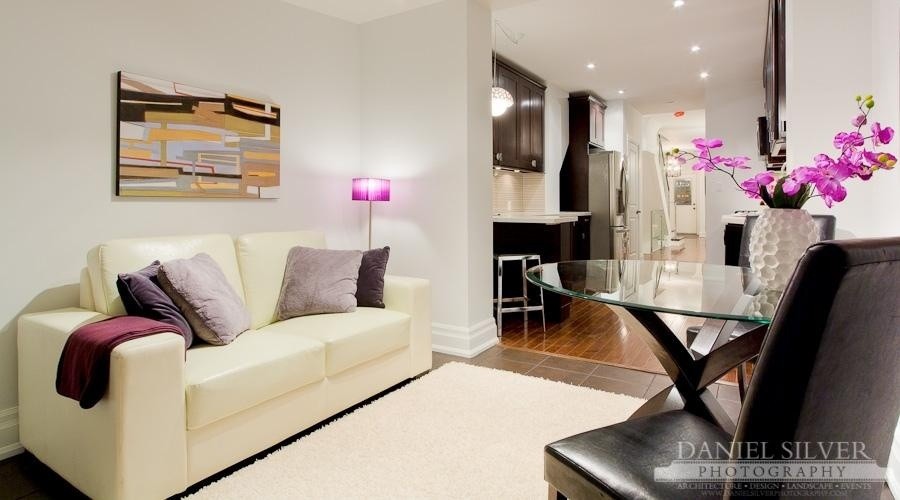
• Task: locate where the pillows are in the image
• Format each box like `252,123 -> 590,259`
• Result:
273,243 -> 390,321
114,253 -> 251,346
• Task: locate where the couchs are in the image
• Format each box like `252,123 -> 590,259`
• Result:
15,227 -> 432,500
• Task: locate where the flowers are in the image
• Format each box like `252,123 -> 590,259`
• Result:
659,94 -> 897,213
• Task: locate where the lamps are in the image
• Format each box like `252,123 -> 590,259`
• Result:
490,21 -> 514,118
351,177 -> 391,249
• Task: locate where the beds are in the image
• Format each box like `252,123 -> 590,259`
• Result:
527,259 -> 783,442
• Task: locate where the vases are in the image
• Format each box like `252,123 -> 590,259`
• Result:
748,207 -> 820,294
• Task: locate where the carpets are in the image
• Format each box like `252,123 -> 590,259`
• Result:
186,360 -> 648,500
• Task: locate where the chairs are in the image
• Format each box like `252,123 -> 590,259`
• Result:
544,238 -> 900,500
687,214 -> 835,399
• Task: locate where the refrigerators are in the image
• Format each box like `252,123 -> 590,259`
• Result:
586,150 -> 628,293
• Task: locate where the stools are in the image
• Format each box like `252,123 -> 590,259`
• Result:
493,250 -> 546,340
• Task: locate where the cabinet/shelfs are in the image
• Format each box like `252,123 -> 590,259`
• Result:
493,61 -> 519,167
517,81 -> 544,170
569,95 -> 606,150
